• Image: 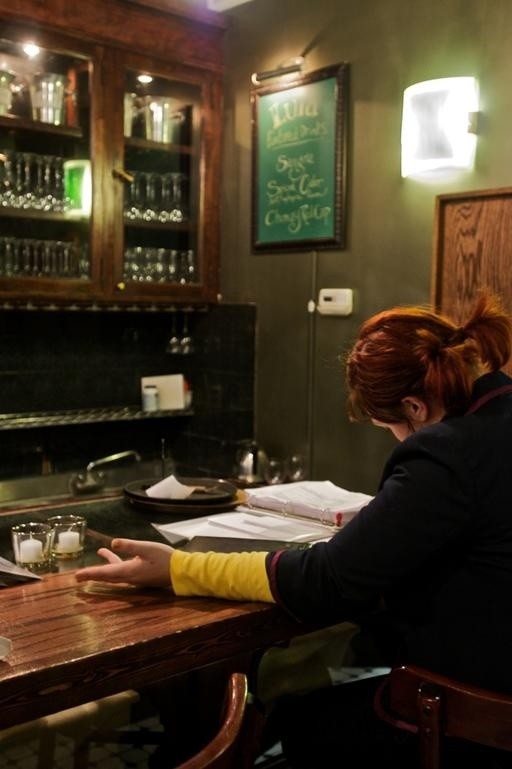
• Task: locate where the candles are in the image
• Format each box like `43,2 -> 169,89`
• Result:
56,526 -> 84,552
18,530 -> 47,563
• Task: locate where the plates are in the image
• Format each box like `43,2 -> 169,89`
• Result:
122,476 -> 238,505
126,486 -> 249,514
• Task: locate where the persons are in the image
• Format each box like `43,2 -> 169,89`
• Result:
69,286 -> 511,769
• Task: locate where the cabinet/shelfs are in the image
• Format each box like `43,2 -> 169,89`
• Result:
0,14 -> 225,308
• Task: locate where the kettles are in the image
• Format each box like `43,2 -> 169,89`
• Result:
236,438 -> 267,484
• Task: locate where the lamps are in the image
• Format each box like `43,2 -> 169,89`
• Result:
401,76 -> 483,185
249,55 -> 304,89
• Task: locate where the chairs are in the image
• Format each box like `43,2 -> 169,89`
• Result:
172,673 -> 248,767
358,662 -> 509,768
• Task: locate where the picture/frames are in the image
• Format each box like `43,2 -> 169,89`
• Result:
249,63 -> 352,256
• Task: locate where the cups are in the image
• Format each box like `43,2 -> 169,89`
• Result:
10,522 -> 54,569
0,236 -> 91,279
123,246 -> 199,286
125,172 -> 186,218
46,514 -> 88,561
0,147 -> 71,212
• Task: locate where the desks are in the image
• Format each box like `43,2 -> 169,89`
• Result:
1,562 -> 350,729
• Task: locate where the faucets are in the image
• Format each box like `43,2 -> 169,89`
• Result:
70,449 -> 143,491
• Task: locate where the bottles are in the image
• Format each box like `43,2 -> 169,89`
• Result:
141,384 -> 160,413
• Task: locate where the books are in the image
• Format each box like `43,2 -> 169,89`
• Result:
240,480 -> 376,528
